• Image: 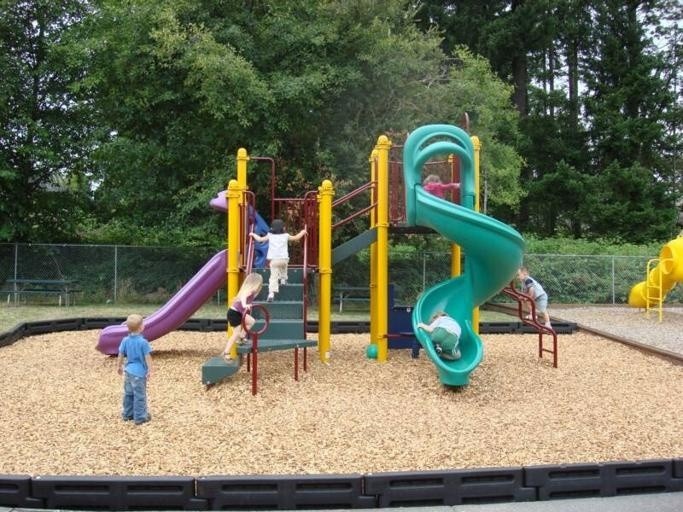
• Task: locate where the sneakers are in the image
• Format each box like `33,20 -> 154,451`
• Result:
266,293 -> 275,301
123,414 -> 150,425
280,280 -> 287,286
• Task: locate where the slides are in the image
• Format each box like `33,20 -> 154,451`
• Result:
95,248 -> 228,356
412,184 -> 525,387
628,238 -> 682,306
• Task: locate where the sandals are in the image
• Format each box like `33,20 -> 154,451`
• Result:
221,351 -> 234,364
237,337 -> 250,345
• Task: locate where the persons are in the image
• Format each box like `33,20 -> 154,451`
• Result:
418,311 -> 461,360
517,266 -> 552,331
249,220 -> 308,302
116,314 -> 152,424
422,175 -> 461,200
220,273 -> 264,364
